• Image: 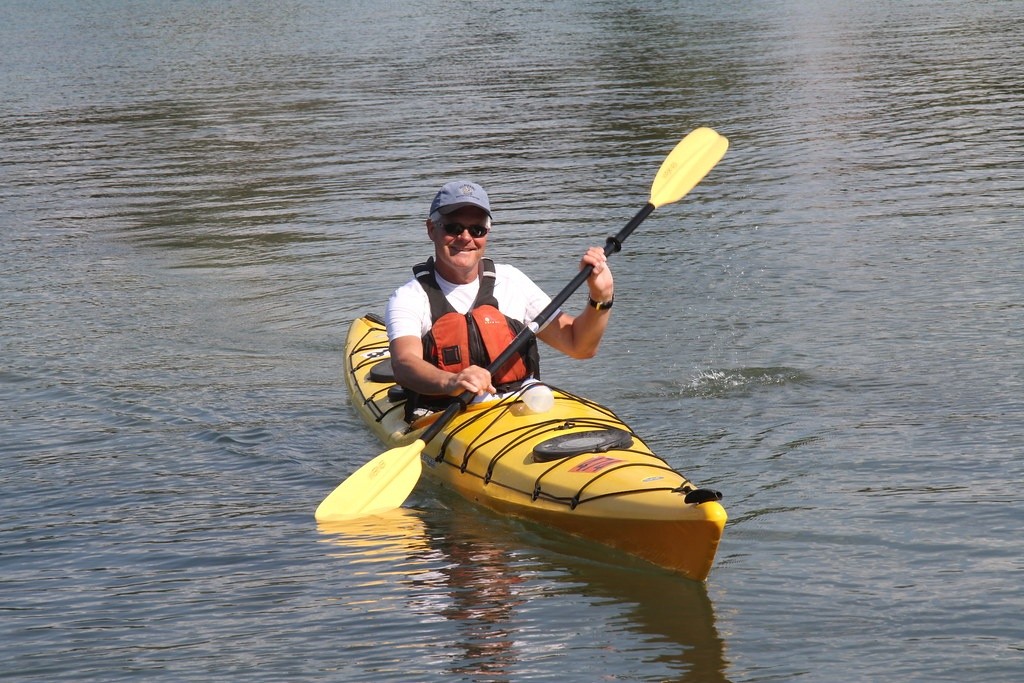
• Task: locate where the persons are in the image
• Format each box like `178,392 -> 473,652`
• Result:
384,181 -> 613,421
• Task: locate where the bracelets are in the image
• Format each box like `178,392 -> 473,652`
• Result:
587,294 -> 614,311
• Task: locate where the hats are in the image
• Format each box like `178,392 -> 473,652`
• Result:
430,180 -> 492,219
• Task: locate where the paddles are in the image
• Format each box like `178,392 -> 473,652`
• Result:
309,122 -> 731,526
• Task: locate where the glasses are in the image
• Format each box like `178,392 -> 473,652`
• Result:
432,221 -> 490,238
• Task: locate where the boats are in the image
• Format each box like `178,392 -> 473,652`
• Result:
346,313 -> 727,579
